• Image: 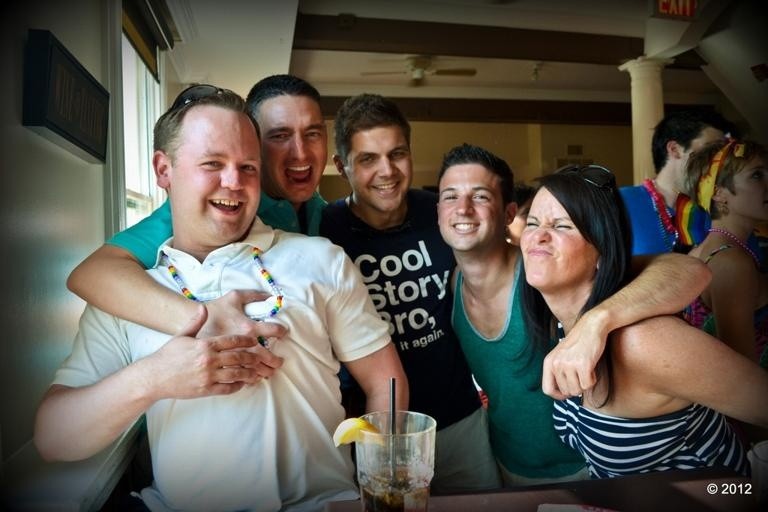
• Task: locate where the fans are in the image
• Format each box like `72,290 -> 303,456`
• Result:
359,55 -> 477,76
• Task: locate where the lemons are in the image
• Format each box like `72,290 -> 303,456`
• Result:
332,417 -> 385,447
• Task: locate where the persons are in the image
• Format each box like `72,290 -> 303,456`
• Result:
62,74 -> 357,388
27,83 -> 412,511
515,160 -> 767,481
613,107 -> 739,329
309,90 -> 507,496
673,138 -> 767,480
502,186 -> 542,245
431,142 -> 716,493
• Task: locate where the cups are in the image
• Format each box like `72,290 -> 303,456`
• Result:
352,412 -> 437,512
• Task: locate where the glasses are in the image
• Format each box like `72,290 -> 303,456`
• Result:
170,84 -> 234,109
550,163 -> 616,188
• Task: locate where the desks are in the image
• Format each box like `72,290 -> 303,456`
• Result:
326,465 -> 768,512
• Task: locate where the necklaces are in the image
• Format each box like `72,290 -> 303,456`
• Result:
661,199 -> 679,239
642,179 -> 678,254
706,225 -> 763,271
160,246 -> 283,352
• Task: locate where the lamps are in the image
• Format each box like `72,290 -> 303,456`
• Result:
412,67 -> 425,79
531,68 -> 540,81
